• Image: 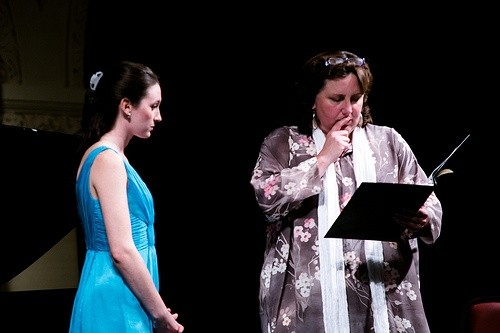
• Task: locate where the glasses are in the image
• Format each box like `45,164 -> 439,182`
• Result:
324,57 -> 366,67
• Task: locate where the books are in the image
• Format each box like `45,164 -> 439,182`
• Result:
324,135 -> 470,242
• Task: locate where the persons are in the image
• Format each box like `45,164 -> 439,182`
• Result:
250,50 -> 443,333
69,61 -> 184,333
